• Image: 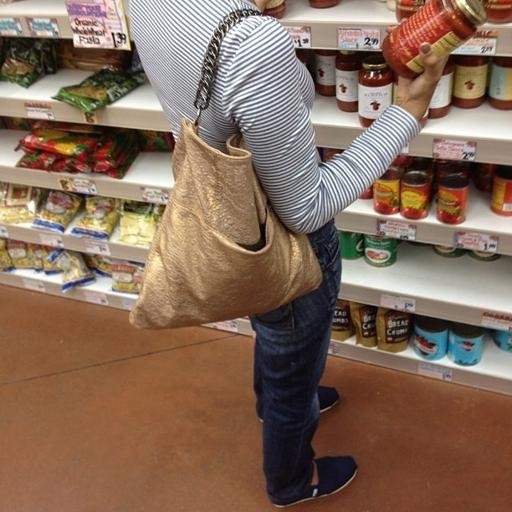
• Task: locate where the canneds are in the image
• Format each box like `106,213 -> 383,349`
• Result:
337,231 -> 499,268
296,48 -> 511,128
329,298 -> 511,366
263,0 -> 511,23
381,2 -> 486,78
317,147 -> 511,224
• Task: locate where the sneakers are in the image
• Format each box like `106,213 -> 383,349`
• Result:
256,383 -> 339,424
270,455 -> 357,508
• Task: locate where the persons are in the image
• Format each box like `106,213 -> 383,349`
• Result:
128,0 -> 450,510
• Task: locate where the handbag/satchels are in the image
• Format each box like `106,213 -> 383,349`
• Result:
128,9 -> 323,331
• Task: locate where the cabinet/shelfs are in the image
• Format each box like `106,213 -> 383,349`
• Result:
0,0 -> 511,397
